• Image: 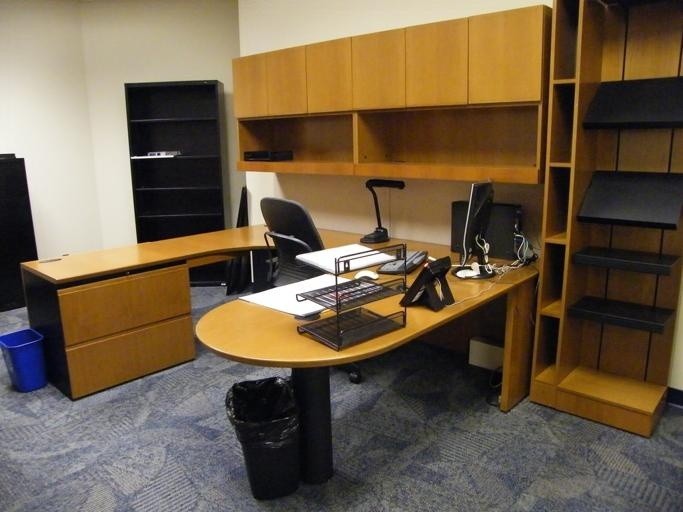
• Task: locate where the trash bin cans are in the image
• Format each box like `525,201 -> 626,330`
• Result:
0,328 -> 49,394
224,383 -> 302,502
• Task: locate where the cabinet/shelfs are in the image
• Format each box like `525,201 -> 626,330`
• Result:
124,79 -> 236,287
229,33 -> 355,177
525,0 -> 582,415
557,0 -> 682,438
351,2 -> 549,184
292,239 -> 407,356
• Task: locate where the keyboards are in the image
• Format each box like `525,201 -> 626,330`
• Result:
377,248 -> 427,273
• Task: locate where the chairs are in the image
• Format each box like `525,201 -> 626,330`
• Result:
260,197 -> 362,384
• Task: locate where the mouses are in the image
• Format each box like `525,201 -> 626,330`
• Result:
353,270 -> 379,280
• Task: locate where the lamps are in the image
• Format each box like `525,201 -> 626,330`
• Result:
357,175 -> 406,244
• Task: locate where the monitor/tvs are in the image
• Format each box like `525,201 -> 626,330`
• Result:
451,182 -> 496,279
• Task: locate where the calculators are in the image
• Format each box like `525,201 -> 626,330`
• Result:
376,249 -> 428,275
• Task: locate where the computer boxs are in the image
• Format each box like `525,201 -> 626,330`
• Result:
451,201 -> 522,259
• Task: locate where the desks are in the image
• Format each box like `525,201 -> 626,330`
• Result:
23,223 -> 537,413
